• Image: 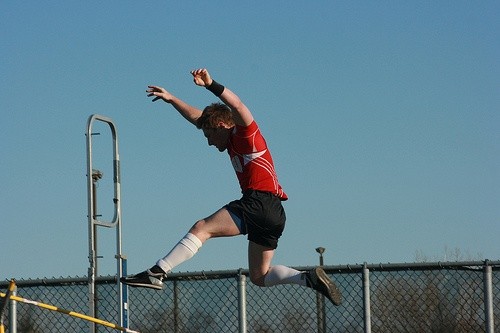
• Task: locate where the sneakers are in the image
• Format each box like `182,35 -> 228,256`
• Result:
305,266 -> 343,306
119,269 -> 167,290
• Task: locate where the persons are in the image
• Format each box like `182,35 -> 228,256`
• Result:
119,69 -> 343,306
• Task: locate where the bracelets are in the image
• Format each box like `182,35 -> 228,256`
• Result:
205,80 -> 225,96
